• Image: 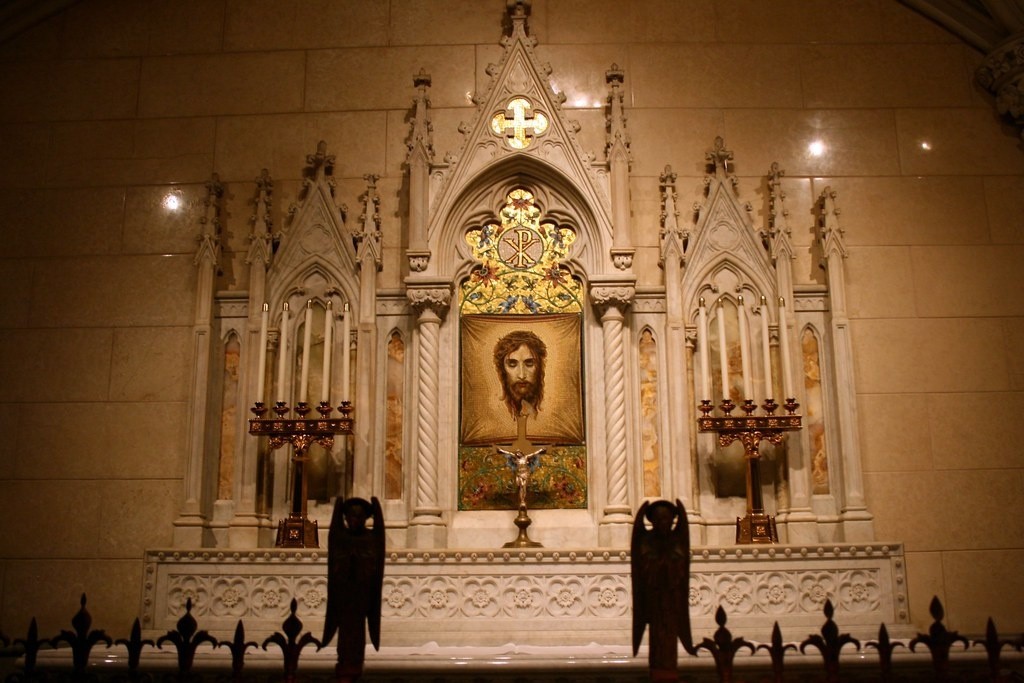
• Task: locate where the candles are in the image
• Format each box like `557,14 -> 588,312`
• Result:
322,300 -> 333,401
738,295 -> 752,399
256,302 -> 270,402
717,298 -> 730,400
277,301 -> 289,401
342,301 -> 351,401
699,296 -> 710,400
299,299 -> 314,402
759,295 -> 774,400
778,296 -> 794,399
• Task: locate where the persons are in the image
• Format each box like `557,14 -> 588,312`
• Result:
498,447 -> 546,502
